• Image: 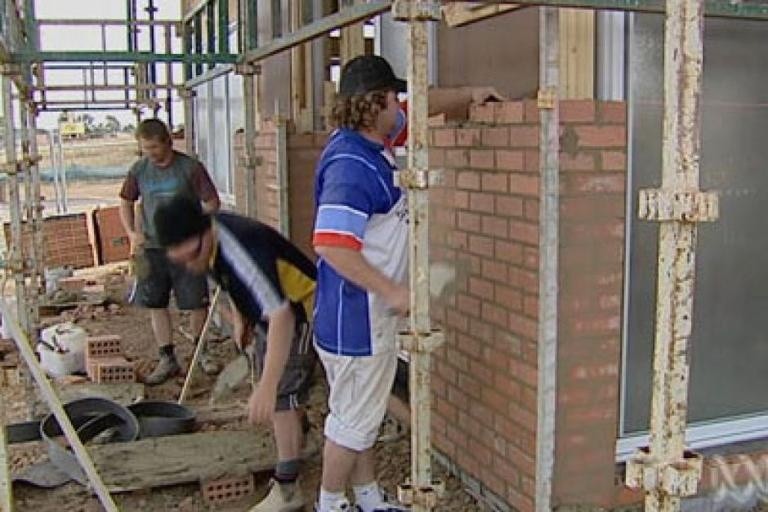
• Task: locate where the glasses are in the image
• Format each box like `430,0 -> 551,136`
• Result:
165,235 -> 203,267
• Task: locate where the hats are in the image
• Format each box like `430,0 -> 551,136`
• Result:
338,55 -> 406,95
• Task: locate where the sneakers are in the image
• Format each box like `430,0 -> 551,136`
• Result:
248,477 -> 305,512
144,356 -> 180,385
353,501 -> 402,512
197,350 -> 218,375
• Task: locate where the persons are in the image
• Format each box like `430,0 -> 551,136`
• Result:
153,195 -> 318,512
302,54 -> 505,510
119,119 -> 225,385
